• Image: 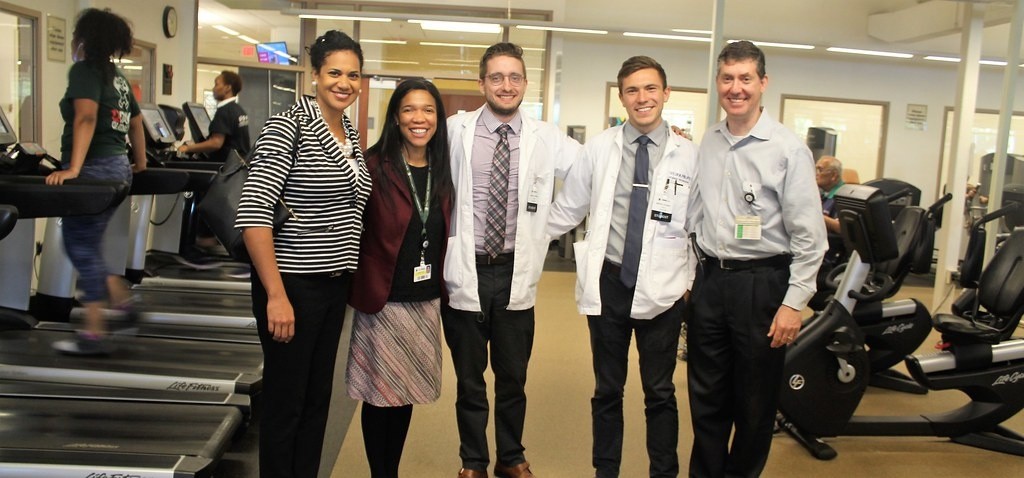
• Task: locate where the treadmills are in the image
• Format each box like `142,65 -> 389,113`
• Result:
0,97 -> 296,478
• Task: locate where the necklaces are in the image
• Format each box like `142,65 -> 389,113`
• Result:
310,97 -> 354,158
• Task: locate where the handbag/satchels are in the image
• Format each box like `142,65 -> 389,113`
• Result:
195,112 -> 300,263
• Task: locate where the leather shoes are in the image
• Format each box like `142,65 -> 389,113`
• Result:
494,459 -> 534,478
457,467 -> 488,478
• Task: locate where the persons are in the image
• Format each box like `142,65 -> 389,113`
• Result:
426,266 -> 430,273
670,41 -> 829,478
546,56 -> 699,478
444,42 -> 693,478
177,70 -> 253,280
361,80 -> 456,478
815,154 -> 846,233
45,7 -> 148,344
232,30 -> 372,478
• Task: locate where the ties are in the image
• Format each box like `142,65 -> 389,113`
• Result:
620,136 -> 651,288
484,125 -> 512,259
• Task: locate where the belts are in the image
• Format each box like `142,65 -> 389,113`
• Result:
475,256 -> 515,264
704,253 -> 785,271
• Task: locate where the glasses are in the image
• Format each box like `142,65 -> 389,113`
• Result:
483,73 -> 526,87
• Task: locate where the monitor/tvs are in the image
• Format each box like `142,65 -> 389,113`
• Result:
255,42 -> 289,66
183,103 -> 212,139
135,103 -> 177,148
0,106 -> 17,146
834,183 -> 899,263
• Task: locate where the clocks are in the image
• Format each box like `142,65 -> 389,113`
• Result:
163,7 -> 177,38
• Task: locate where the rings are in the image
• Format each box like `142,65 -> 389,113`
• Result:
787,336 -> 793,340
